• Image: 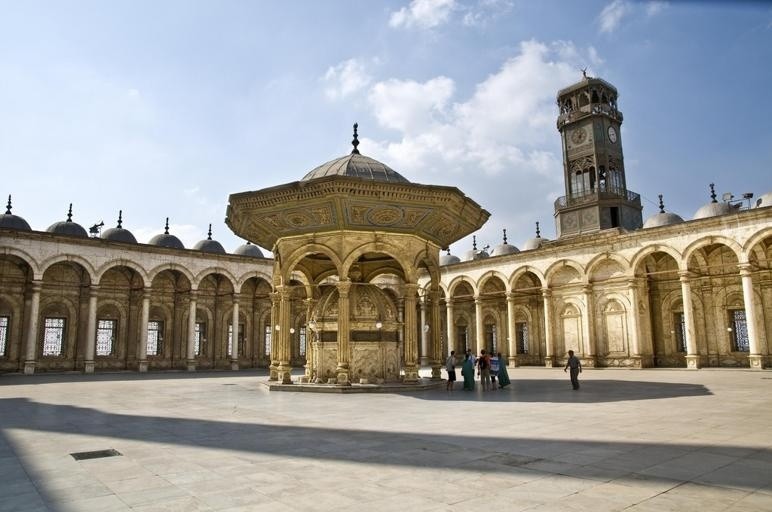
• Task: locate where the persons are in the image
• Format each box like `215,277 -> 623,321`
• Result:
563,350 -> 582,391
445,349 -> 511,392
